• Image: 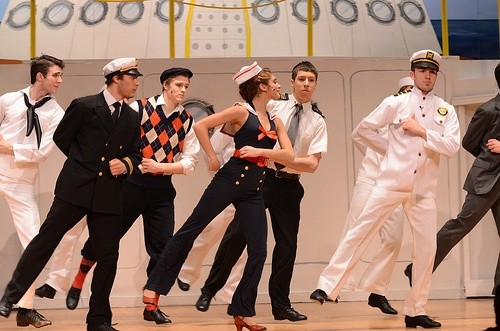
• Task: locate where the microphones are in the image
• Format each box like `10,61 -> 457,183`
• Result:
257,78 -> 277,92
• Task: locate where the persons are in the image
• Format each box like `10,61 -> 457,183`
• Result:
0,57 -> 140,331
65,67 -> 199,325
404,58 -> 500,331
308,48 -> 461,330
143,60 -> 328,331
325,77 -> 414,316
0,55 -> 65,328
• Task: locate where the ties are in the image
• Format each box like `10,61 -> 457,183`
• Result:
110,102 -> 122,126
23,93 -> 51,151
274,104 -> 303,170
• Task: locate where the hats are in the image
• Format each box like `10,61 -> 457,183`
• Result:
409,49 -> 443,73
232,61 -> 262,85
398,76 -> 414,93
102,57 -> 144,79
159,67 -> 194,85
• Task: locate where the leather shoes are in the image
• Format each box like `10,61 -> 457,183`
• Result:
0,295 -> 13,318
404,315 -> 441,328
177,278 -> 190,291
87,323 -> 120,331
66,285 -> 82,310
404,263 -> 413,288
273,308 -> 308,322
34,284 -> 57,300
367,293 -> 399,315
16,309 -> 52,328
309,289 -> 339,306
142,307 -> 172,325
195,294 -> 212,312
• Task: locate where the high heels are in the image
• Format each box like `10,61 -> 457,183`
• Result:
142,286 -> 156,305
234,316 -> 267,331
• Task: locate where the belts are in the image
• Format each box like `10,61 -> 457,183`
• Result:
269,169 -> 299,180
